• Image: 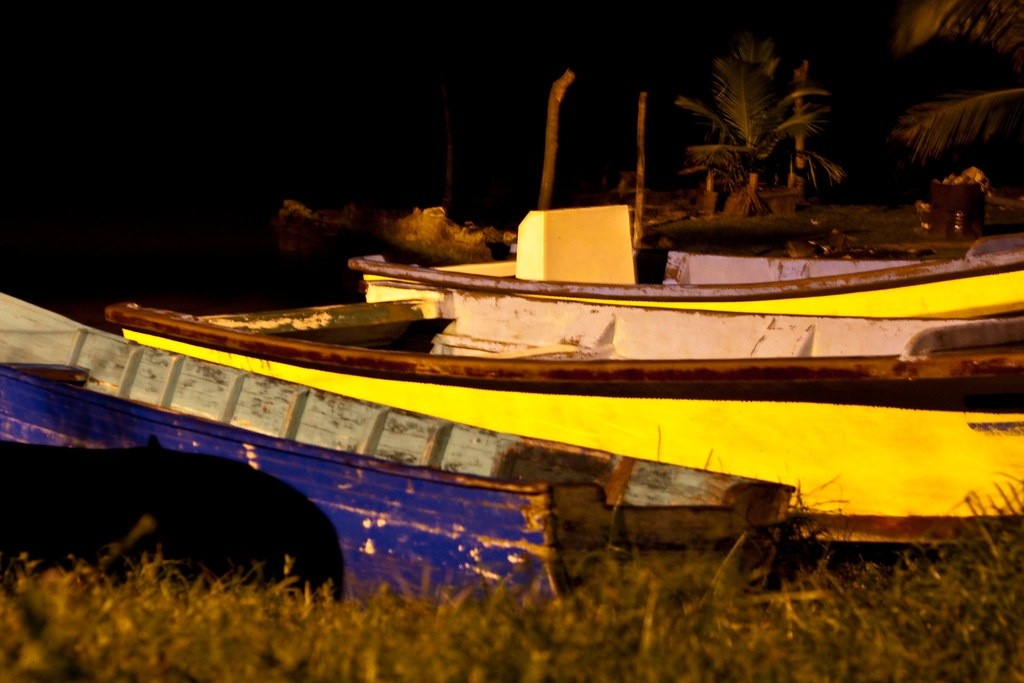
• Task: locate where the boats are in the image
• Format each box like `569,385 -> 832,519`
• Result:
0,203 -> 1024,597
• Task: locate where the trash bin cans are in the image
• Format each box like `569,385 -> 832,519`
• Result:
931,179 -> 986,242
